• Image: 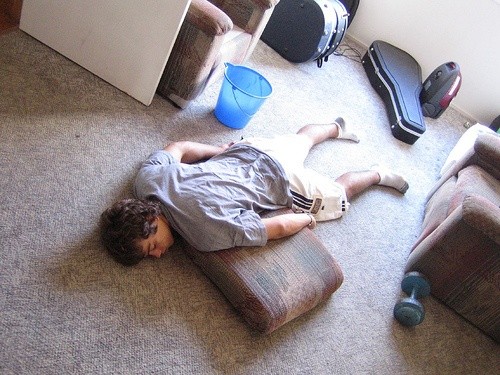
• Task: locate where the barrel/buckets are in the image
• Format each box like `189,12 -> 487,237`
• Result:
215,61 -> 273,130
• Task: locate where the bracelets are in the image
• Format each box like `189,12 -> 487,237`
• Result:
306,213 -> 313,228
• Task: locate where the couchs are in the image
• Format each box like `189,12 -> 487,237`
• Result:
403,123 -> 500,336
156,0 -> 280,110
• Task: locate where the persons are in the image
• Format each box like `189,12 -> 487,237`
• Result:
99,117 -> 409,267
